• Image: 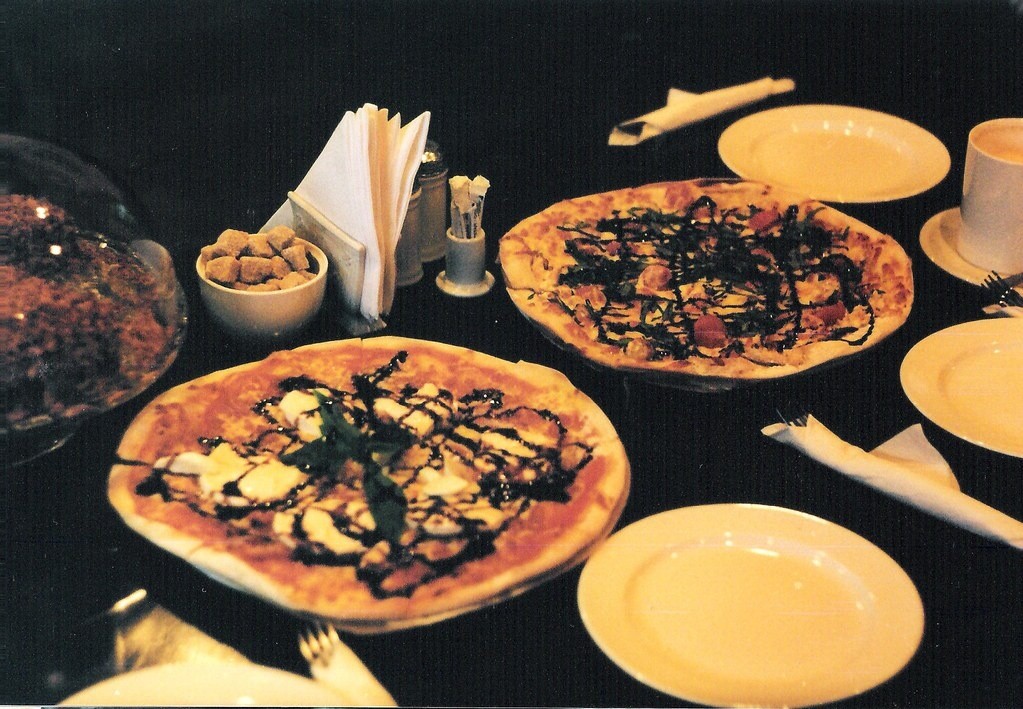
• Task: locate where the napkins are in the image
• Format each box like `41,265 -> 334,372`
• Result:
257,100 -> 432,321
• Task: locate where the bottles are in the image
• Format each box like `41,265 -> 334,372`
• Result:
395,138 -> 449,286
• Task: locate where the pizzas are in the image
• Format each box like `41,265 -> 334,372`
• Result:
501,179 -> 915,368
105,337 -> 633,634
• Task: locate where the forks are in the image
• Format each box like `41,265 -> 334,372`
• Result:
774,397 -> 810,430
294,618 -> 339,662
613,121 -> 647,138
980,270 -> 1023,308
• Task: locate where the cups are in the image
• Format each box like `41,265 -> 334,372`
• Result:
957,118 -> 1023,276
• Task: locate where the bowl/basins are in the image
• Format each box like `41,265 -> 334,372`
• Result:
0,228 -> 188,469
196,231 -> 328,343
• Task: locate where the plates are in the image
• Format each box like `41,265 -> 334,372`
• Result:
577,502 -> 925,709
919,207 -> 1023,297
899,317 -> 1023,461
717,104 -> 952,205
55,663 -> 401,707
